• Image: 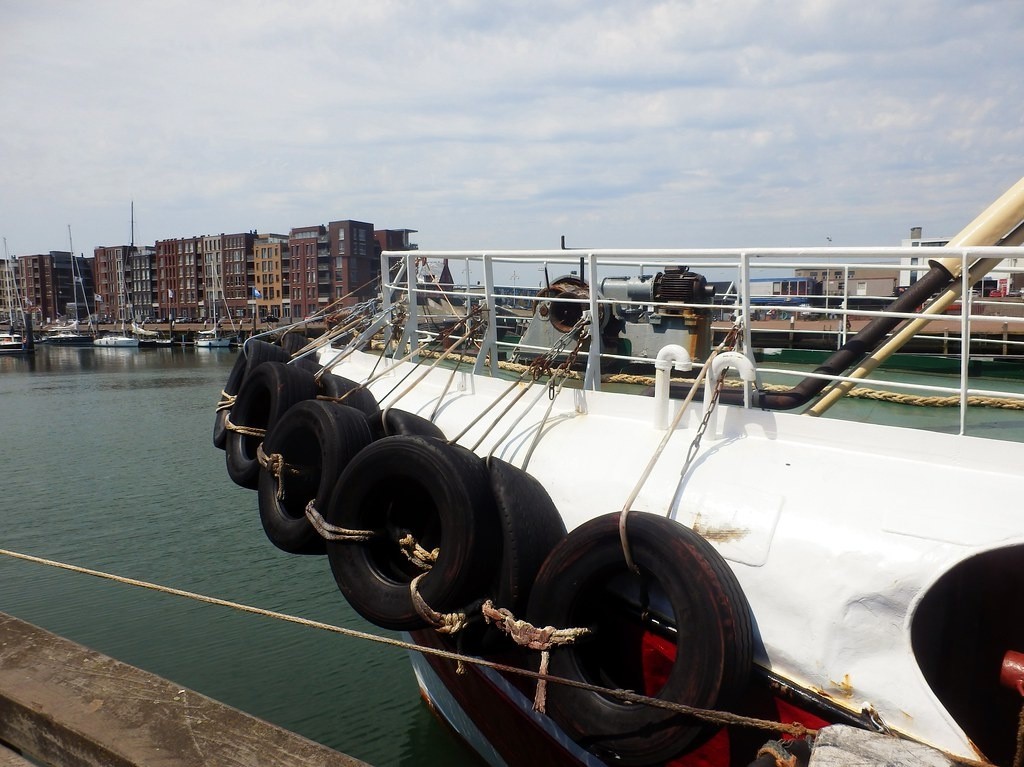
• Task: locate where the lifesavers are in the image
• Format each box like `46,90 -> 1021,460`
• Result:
212,332 -> 758,767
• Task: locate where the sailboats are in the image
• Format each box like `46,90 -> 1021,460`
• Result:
0,225 -> 240,350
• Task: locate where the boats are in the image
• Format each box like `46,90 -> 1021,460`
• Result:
210,245 -> 1023,767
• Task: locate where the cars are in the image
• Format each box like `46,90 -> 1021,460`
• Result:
67,319 -> 81,325
175,316 -> 185,324
264,315 -> 280,322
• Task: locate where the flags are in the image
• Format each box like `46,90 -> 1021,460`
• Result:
253,287 -> 261,298
95,294 -> 102,302
25,297 -> 32,306
169,289 -> 173,297
75,277 -> 83,282
